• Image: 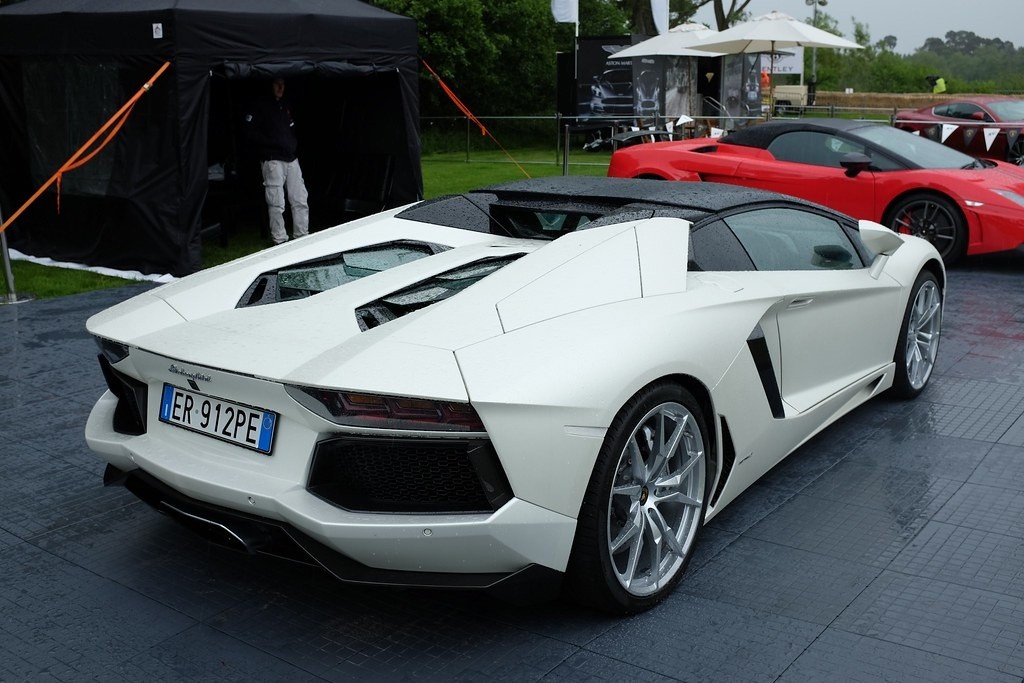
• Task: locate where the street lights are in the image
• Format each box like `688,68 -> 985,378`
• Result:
805,0 -> 828,102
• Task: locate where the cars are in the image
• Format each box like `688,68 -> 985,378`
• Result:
590,68 -> 635,118
636,71 -> 660,117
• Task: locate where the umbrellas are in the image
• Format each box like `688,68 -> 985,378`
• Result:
606,23 -> 729,116
685,13 -> 867,109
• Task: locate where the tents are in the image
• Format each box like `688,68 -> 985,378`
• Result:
0,0 -> 424,282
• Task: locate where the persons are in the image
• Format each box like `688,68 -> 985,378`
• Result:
242,77 -> 309,243
761,71 -> 770,87
806,74 -> 824,106
925,76 -> 947,94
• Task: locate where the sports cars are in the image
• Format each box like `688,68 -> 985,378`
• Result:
894,94 -> 1024,162
608,119 -> 1024,267
82,177 -> 947,609
